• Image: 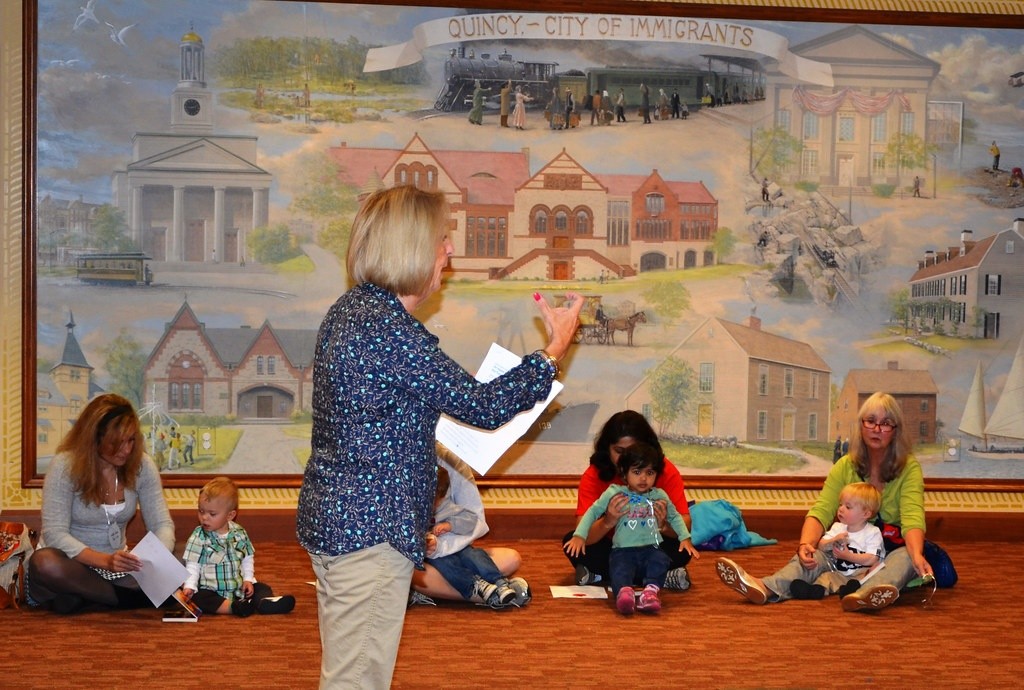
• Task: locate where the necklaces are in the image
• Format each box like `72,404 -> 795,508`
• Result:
98,470 -> 119,531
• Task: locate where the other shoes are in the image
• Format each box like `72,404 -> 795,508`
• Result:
841,585 -> 898,611
715,557 -> 767,605
790,579 -> 824,599
258,595 -> 295,614
232,599 -> 254,617
839,579 -> 861,598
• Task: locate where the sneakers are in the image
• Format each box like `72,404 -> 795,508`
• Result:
637,589 -> 661,612
616,587 -> 635,614
663,567 -> 691,590
472,579 -> 497,602
496,584 -> 516,605
487,577 -> 531,610
576,565 -> 602,586
407,590 -> 438,607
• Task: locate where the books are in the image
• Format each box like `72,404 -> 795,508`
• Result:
162,590 -> 202,623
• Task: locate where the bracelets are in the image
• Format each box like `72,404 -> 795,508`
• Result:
599,516 -> 611,529
797,543 -> 811,556
533,349 -> 562,379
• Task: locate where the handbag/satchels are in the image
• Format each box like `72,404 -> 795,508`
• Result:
875,519 -> 906,552
0,521 -> 38,609
924,540 -> 957,588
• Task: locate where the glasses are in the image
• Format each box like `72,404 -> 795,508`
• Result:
862,418 -> 896,432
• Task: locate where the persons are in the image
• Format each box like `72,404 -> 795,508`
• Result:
294,186 -> 585,690
24,394 -> 175,615
408,440 -> 533,611
562,410 -> 699,614
715,391 -> 934,612
180,477 -> 295,618
